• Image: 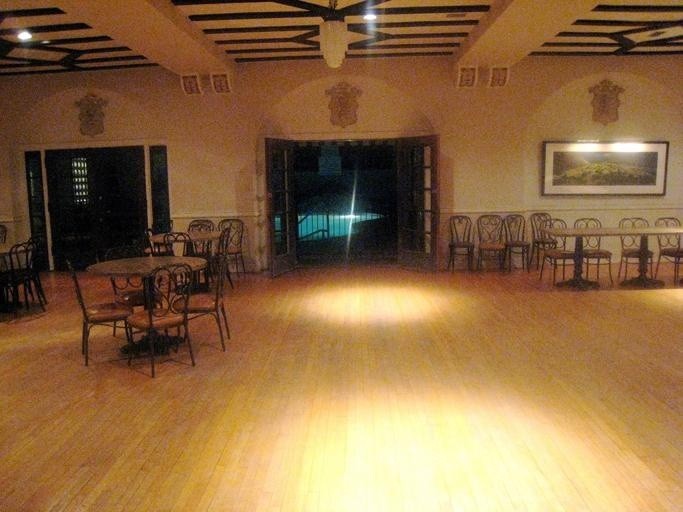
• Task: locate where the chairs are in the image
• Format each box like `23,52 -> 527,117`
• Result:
144,218 -> 246,295
654,217 -> 683,283
65,257 -> 134,366
173,256 -> 231,351
0,224 -> 49,321
127,262 -> 195,378
104,245 -> 164,340
617,217 -> 654,284
447,213 -> 557,273
539,219 -> 576,285
574,218 -> 614,286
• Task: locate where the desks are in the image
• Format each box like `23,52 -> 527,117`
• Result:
86,256 -> 207,356
539,226 -> 683,291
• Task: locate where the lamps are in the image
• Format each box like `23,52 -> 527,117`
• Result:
317,0 -> 350,70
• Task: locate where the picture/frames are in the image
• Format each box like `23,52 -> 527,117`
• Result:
542,140 -> 669,196
